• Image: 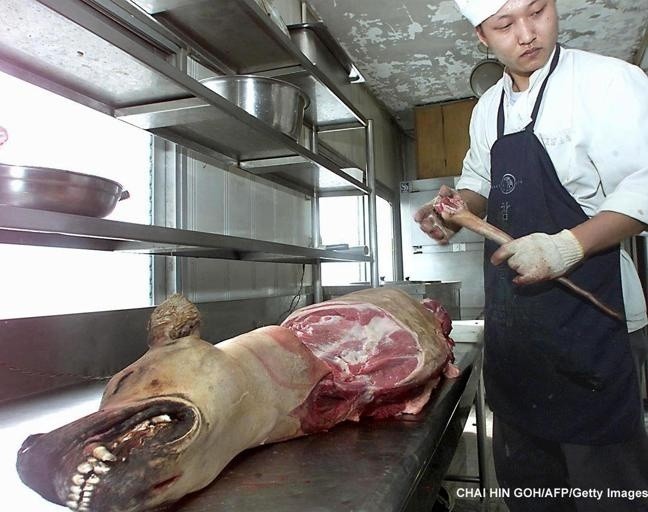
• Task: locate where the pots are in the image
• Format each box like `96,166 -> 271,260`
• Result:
1,163 -> 129,219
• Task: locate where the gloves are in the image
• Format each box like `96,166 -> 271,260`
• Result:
414,184 -> 468,245
491,228 -> 584,286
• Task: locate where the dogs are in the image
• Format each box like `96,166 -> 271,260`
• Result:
16,286 -> 462,512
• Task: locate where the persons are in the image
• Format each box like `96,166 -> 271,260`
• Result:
412,0 -> 648,512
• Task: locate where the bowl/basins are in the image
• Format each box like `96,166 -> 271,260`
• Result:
198,74 -> 310,143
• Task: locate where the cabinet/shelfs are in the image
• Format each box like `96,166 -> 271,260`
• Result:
0,0 -> 380,400
413,97 -> 478,180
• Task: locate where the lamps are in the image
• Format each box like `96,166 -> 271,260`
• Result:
469,42 -> 508,98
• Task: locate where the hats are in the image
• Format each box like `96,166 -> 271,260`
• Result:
455,0 -> 508,28
